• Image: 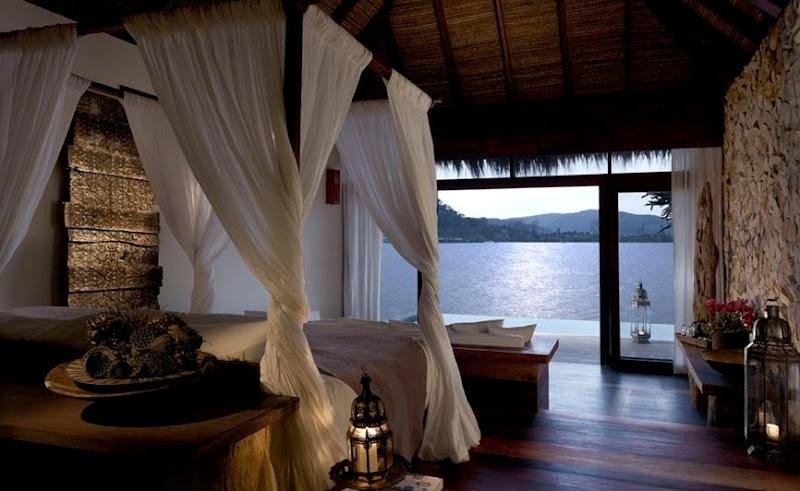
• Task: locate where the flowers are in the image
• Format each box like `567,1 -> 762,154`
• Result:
703,297 -> 755,337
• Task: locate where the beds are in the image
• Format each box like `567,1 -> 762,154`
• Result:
0,0 -> 481,491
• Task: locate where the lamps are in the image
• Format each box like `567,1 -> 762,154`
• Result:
744,298 -> 800,465
630,282 -> 652,343
328,373 -> 408,491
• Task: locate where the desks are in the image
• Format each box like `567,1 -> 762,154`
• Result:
0,374 -> 299,491
675,333 -> 744,427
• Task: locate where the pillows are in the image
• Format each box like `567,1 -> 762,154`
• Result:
307,320 -> 536,348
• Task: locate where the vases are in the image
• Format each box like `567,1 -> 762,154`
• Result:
710,330 -> 749,350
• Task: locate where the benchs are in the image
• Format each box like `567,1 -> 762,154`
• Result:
452,336 -> 558,413
